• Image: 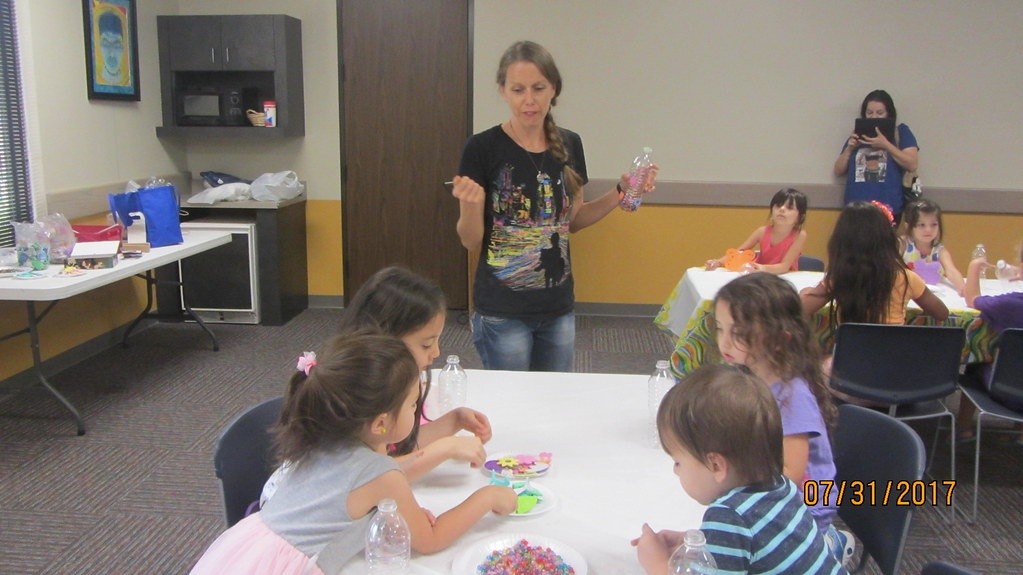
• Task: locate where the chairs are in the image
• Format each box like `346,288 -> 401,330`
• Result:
825,322 -> 971,528
934,329 -> 1023,524
215,397 -> 310,528
815,404 -> 922,573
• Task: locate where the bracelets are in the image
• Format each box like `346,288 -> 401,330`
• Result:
617,184 -> 626,196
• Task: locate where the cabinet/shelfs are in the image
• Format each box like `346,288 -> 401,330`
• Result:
155,193 -> 310,326
155,13 -> 306,140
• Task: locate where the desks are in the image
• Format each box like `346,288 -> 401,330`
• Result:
341,364 -> 723,574
1,230 -> 237,436
655,268 -> 1023,470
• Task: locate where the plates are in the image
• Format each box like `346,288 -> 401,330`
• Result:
14,273 -> 48,279
926,283 -> 946,291
482,451 -> 550,477
0,266 -> 33,275
55,271 -> 88,277
452,533 -> 588,575
488,481 -> 557,516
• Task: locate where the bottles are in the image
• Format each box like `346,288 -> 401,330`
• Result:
621,146 -> 653,216
667,529 -> 718,575
263,100 -> 277,127
439,355 -> 468,439
366,498 -> 410,574
970,244 -> 987,292
647,360 -> 675,448
740,264 -> 753,276
995,260 -> 1021,293
544,178 -> 550,193
545,196 -> 552,218
17,239 -> 48,270
511,185 -> 524,210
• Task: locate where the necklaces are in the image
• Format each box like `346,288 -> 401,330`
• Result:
510,120 -> 546,183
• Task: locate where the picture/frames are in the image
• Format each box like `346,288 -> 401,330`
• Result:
81,0 -> 140,101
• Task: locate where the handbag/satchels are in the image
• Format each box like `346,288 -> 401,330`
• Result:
107,184 -> 183,247
247,171 -> 303,202
895,122 -> 922,210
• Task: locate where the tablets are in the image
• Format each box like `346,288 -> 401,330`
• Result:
855,118 -> 895,144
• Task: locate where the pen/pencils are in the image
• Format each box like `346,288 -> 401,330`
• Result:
444,181 -> 454,186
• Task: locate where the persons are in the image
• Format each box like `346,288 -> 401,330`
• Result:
946,247 -> 1023,446
896,198 -> 966,296
339,265 -> 491,483
633,364 -> 851,575
455,40 -> 659,373
190,324 -> 519,575
713,272 -> 855,568
835,90 -> 920,227
800,201 -> 949,409
707,188 -> 808,274
